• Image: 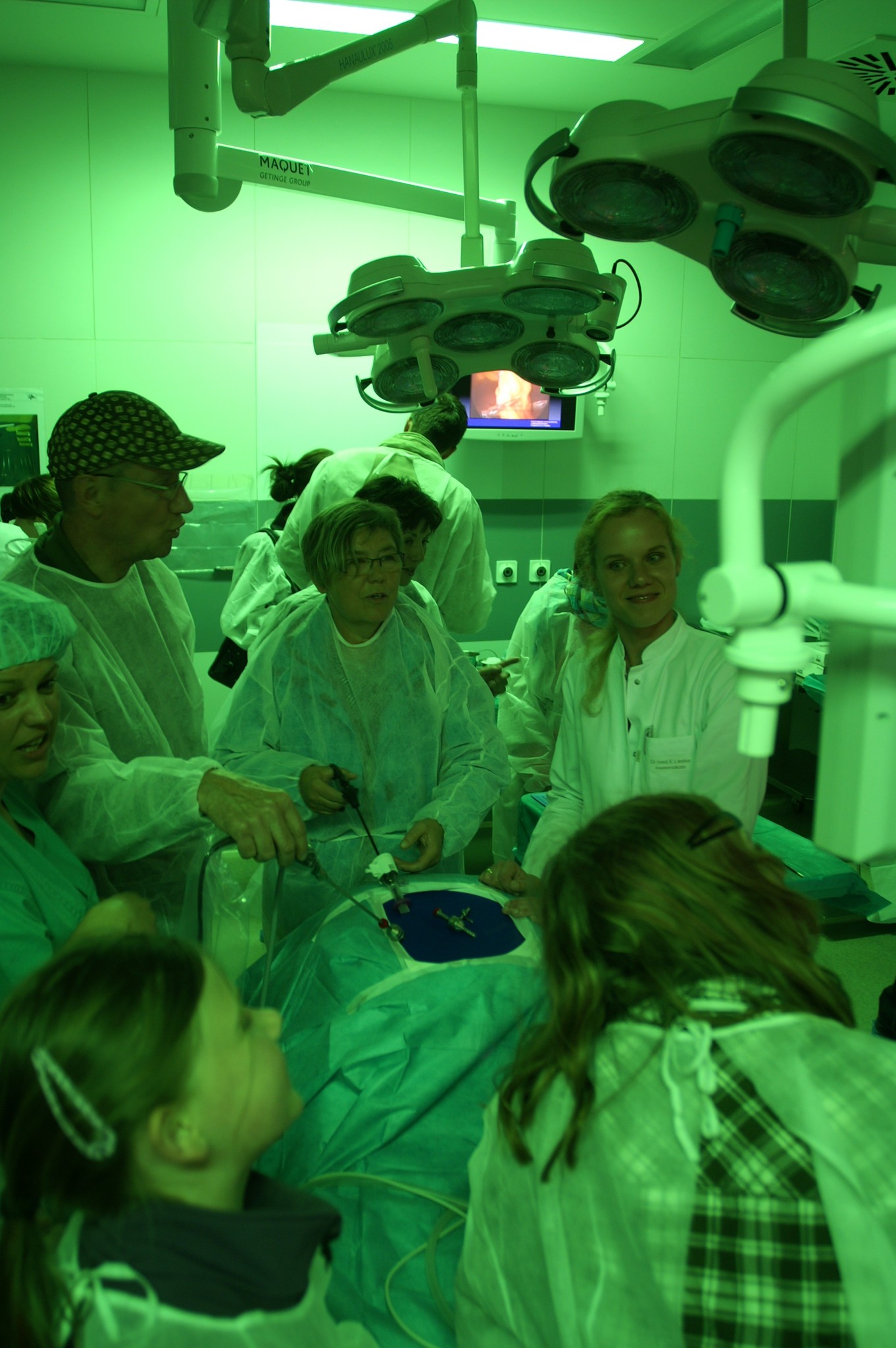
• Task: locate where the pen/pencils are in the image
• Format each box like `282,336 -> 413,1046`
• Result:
784,863 -> 805,878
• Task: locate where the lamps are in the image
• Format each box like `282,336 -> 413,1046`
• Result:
311,31 -> 627,416
523,0 -> 896,340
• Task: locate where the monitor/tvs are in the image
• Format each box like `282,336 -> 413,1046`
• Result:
447,370 -> 583,442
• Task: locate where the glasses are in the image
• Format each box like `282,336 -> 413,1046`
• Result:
342,552 -> 407,578
90,471 -> 189,498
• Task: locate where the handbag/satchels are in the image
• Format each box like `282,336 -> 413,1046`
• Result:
207,635 -> 248,688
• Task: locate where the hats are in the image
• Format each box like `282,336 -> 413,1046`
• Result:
47,390 -> 224,474
1,581 -> 77,668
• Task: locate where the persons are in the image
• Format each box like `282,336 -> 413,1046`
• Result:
449,791 -> 896,1348
208,390 -> 745,938
0,929 -> 385,1347
0,390 -> 310,932
0,579 -> 115,1003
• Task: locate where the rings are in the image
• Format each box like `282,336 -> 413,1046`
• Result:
487,868 -> 493,874
